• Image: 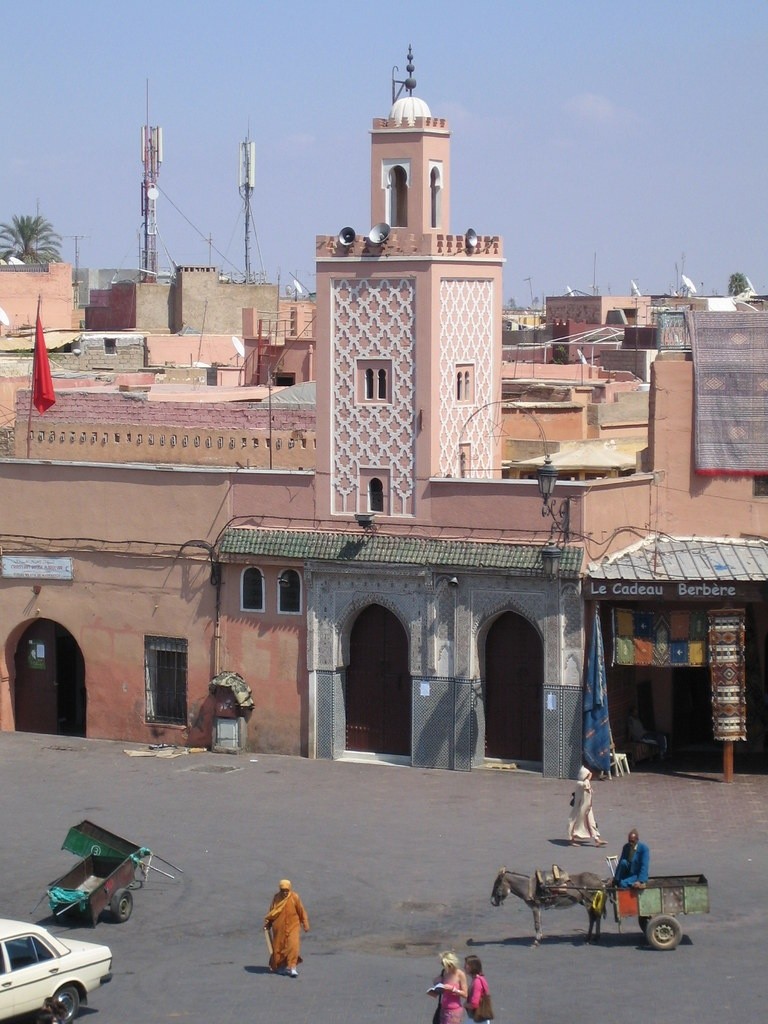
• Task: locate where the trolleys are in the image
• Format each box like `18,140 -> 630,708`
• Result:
60,820 -> 183,879
28,853 -> 137,923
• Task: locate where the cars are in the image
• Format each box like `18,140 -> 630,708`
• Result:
0,919 -> 114,1024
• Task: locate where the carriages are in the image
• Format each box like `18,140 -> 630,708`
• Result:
489,864 -> 711,952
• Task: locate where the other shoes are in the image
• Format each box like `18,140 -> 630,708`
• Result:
595,841 -> 607,845
288,970 -> 298,976
267,963 -> 276,971
571,842 -> 580,847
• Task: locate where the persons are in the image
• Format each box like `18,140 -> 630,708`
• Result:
611,831 -> 649,889
569,765 -> 607,847
263,880 -> 309,976
427,951 -> 468,1024
462,955 -> 490,1024
626,708 -> 667,760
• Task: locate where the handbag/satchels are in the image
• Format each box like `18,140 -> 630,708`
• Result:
472,995 -> 494,1022
432,1003 -> 439,1024
570,792 -> 575,807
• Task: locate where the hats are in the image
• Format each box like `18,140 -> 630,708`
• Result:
279,880 -> 291,890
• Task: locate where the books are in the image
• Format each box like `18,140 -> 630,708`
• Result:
426,983 -> 445,998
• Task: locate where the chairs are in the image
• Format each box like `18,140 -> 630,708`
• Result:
601,753 -> 630,777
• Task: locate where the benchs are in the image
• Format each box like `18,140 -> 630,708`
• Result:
616,741 -> 656,767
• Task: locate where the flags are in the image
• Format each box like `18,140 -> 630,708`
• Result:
33,317 -> 55,414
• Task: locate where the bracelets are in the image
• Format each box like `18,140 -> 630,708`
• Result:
452,988 -> 456,992
457,990 -> 460,995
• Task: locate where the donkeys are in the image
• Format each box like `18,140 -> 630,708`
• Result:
491,866 -> 607,946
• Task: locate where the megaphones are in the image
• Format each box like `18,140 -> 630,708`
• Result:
465,228 -> 478,246
447,577 -> 460,589
339,227 -> 356,246
369,222 -> 390,244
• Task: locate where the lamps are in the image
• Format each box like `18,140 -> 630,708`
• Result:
540,517 -> 569,582
537,453 -> 571,517
355,512 -> 376,529
